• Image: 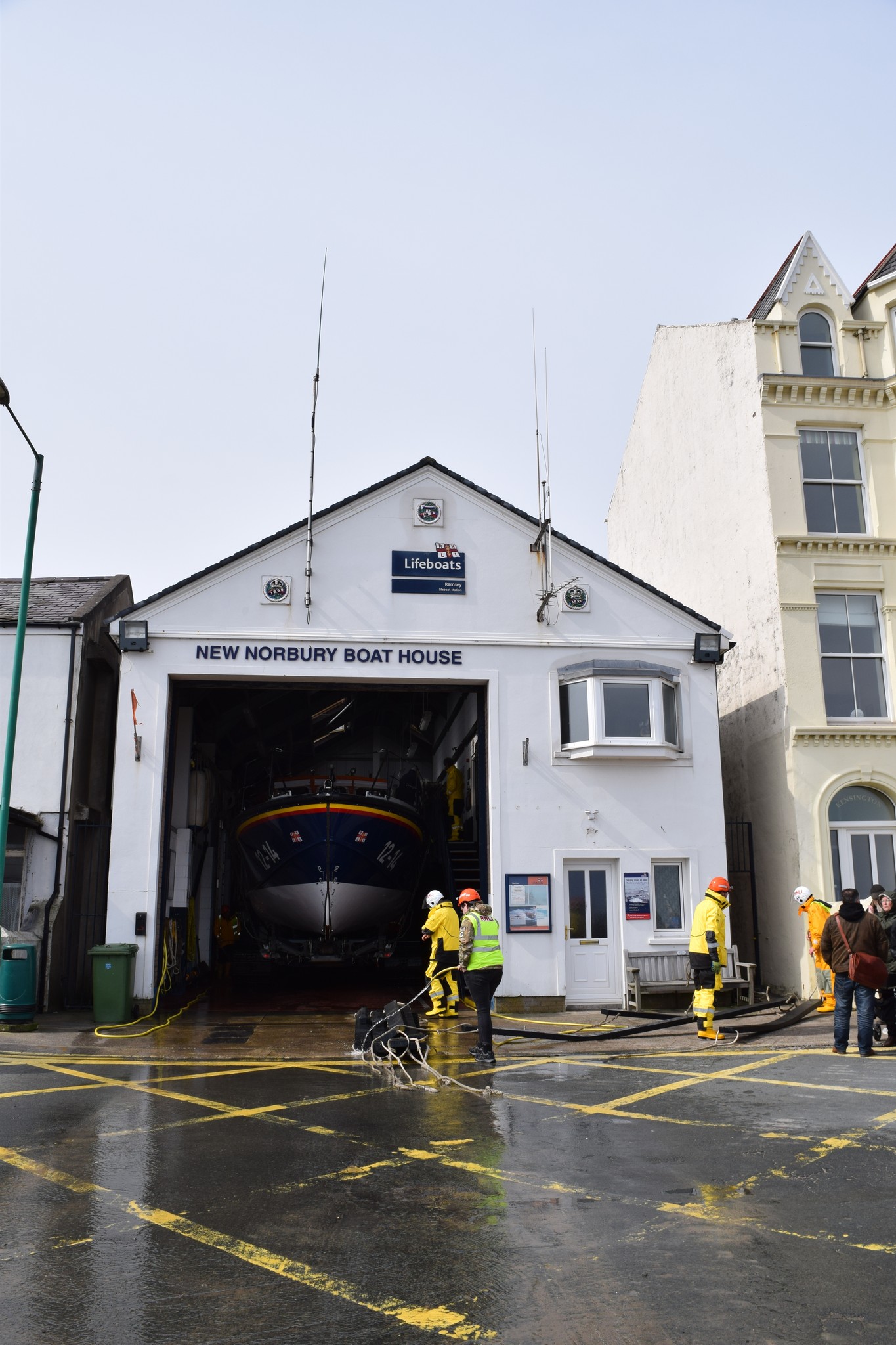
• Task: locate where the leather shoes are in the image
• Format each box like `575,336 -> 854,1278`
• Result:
881,1036 -> 896,1047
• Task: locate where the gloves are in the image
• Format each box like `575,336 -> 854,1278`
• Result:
711,961 -> 721,975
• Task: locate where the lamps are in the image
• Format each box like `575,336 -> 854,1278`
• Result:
119,620 -> 149,653
407,691 -> 418,757
419,692 -> 433,730
694,633 -> 721,663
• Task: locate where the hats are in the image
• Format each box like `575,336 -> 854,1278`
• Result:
870,884 -> 886,899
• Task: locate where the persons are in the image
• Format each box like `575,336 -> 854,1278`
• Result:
689,877 -> 734,1039
794,886 -> 836,1012
820,889 -> 888,1057
422,890 -> 461,1019
458,888 -> 503,1062
866,884 -> 896,1048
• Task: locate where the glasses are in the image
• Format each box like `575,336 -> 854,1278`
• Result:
882,899 -> 891,903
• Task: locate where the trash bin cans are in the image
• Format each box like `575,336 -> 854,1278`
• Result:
86,944 -> 139,1025
0,944 -> 38,1033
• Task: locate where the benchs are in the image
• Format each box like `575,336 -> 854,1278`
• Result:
624,945 -> 757,1009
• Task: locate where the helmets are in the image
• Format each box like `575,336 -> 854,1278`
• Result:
709,877 -> 730,892
457,888 -> 481,906
794,886 -> 812,905
422,890 -> 444,909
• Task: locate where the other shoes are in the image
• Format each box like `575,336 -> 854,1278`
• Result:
697,1029 -> 725,1040
439,1011 -> 459,1019
882,1028 -> 888,1035
817,1005 -> 835,1012
832,1046 -> 846,1054
713,1029 -> 719,1033
860,1049 -> 875,1057
425,1007 -> 447,1016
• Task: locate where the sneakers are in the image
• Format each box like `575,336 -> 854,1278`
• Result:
469,1046 -> 484,1056
474,1051 -> 496,1063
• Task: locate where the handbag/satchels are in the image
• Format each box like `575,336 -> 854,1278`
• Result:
847,950 -> 888,990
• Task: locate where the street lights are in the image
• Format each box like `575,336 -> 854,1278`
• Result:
0,376 -> 45,912
